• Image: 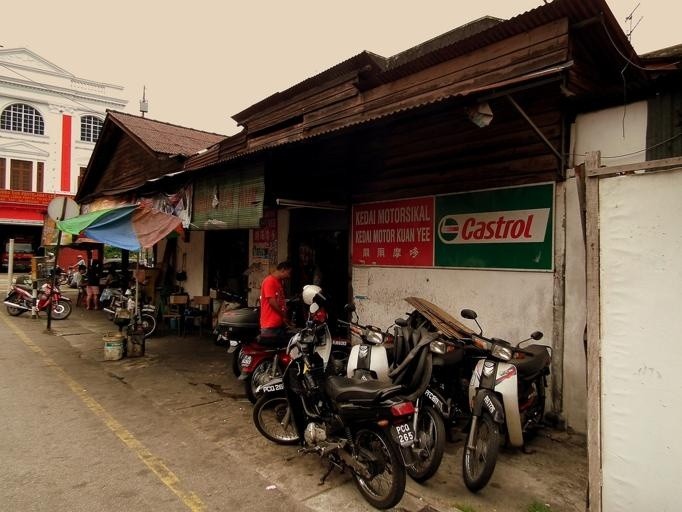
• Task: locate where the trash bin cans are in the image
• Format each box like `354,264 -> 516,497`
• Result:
127,332 -> 145,358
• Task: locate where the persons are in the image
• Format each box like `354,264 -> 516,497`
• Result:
258,260 -> 293,335
69,254 -> 131,310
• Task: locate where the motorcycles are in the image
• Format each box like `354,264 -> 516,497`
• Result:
99,278 -> 156,338
3,268 -> 72,320
58,264 -> 87,284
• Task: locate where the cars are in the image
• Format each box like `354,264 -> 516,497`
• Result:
3,240 -> 33,273
68,258 -> 144,291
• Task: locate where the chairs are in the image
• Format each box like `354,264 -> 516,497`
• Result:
160,293 -> 212,340
